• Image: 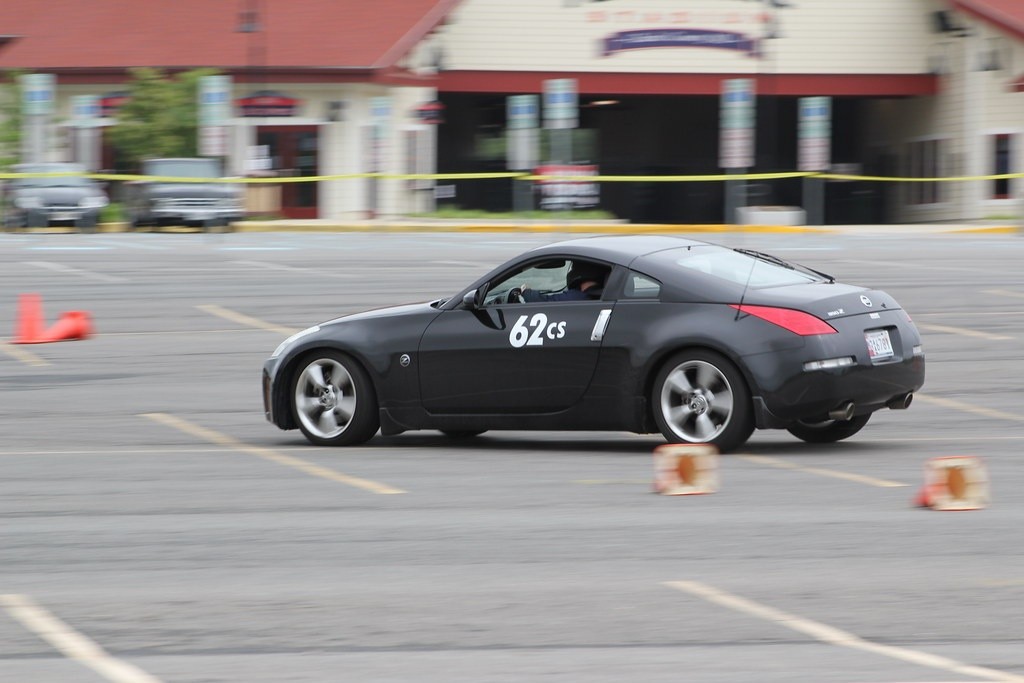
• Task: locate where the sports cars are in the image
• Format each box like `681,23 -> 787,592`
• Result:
261,233 -> 925,453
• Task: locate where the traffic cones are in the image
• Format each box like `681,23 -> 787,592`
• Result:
42,308 -> 94,342
649,442 -> 720,497
12,290 -> 46,344
910,455 -> 990,513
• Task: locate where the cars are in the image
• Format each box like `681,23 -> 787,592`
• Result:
0,163 -> 109,228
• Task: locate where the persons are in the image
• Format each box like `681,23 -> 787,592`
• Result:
521,261 -> 607,303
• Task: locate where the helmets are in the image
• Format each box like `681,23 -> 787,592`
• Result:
566,260 -> 611,290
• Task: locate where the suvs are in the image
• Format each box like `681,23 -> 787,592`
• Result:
126,157 -> 246,229
